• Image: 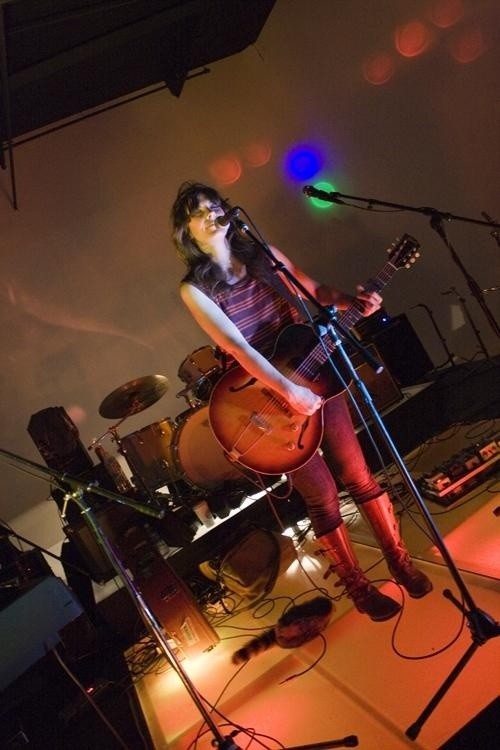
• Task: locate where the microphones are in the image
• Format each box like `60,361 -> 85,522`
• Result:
214,206 -> 240,230
301,185 -> 344,205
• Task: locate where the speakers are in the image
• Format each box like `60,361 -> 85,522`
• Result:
26,406 -> 95,484
365,313 -> 438,388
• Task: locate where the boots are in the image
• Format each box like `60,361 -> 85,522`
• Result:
356,489 -> 433,599
314,521 -> 402,623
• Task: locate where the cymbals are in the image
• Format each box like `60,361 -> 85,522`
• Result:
98,374 -> 167,419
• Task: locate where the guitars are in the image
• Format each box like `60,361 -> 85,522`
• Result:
209,233 -> 418,475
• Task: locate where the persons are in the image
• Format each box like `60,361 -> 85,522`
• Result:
170,176 -> 433,622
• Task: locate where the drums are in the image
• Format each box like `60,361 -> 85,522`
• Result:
177,344 -> 222,400
120,417 -> 181,491
171,405 -> 248,494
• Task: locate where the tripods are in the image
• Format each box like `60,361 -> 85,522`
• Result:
0,448 -> 359,750
233,218 -> 500,743
